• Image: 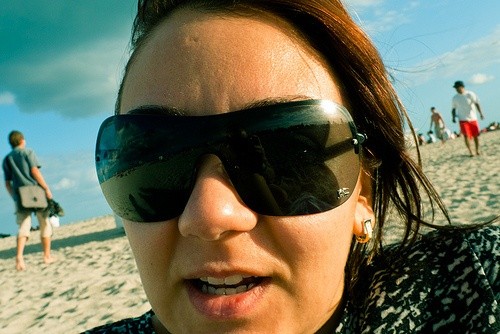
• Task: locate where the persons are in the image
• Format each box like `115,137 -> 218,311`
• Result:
222,116 -> 340,216
79,0 -> 500,334
2,130 -> 54,269
417,107 -> 500,144
451,80 -> 484,157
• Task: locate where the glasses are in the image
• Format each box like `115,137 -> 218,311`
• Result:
95,99 -> 369,222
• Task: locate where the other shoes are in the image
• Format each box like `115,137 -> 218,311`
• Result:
49,199 -> 56,217
56,202 -> 64,217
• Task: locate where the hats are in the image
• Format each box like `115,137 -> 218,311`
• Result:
453,81 -> 464,88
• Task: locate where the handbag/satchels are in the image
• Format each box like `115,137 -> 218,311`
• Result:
19,186 -> 48,211
